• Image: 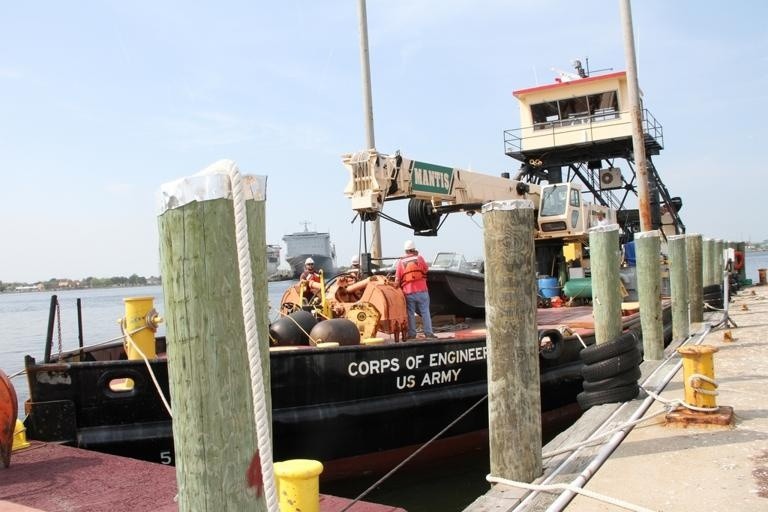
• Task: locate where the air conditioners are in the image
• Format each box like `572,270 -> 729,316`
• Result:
600,168 -> 621,189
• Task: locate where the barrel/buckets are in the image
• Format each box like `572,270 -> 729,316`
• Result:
0,368 -> 18,470
625,241 -> 636,266
538,276 -> 559,298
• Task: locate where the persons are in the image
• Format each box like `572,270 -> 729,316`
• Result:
299,257 -> 326,305
393,240 -> 437,341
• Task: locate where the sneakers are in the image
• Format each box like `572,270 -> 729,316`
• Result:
408,333 -> 439,339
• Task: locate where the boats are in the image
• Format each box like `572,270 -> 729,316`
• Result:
282,221 -> 337,272
25,60 -> 686,475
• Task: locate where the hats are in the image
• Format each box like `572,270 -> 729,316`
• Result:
406,240 -> 415,250
304,258 -> 314,265
